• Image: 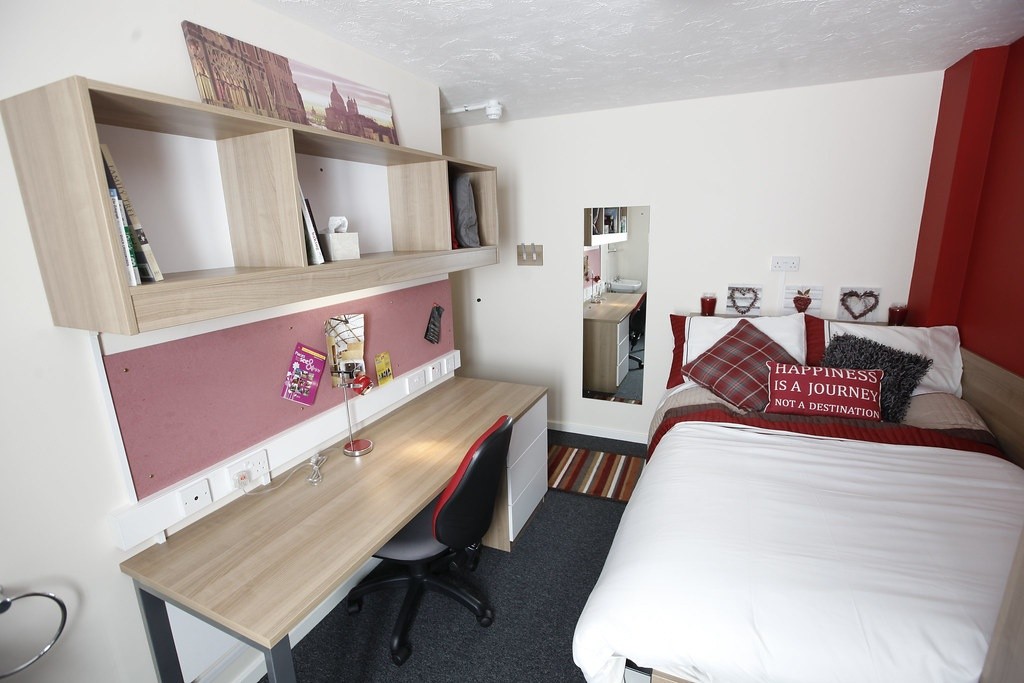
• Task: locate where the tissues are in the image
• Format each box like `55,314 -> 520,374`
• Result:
319,216 -> 361,261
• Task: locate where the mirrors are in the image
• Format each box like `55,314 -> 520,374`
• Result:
581,205 -> 648,405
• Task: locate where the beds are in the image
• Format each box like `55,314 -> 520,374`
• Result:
570,311 -> 1024,683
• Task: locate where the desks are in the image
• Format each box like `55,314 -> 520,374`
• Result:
582,292 -> 641,393
121,375 -> 549,683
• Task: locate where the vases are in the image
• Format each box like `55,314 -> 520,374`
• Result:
701,298 -> 714,316
888,307 -> 905,325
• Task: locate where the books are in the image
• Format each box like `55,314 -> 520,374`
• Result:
99,141 -> 164,287
298,180 -> 325,265
604,208 -> 627,235
281,342 -> 327,407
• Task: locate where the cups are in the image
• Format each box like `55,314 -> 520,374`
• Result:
701,297 -> 716,316
888,306 -> 906,326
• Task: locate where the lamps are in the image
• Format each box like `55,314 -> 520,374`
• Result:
483,101 -> 503,120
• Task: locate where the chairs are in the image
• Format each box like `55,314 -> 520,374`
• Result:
348,412 -> 514,665
628,293 -> 646,368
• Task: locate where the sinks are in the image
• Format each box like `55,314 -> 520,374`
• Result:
607,279 -> 642,293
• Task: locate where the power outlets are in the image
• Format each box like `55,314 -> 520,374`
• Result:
771,255 -> 800,272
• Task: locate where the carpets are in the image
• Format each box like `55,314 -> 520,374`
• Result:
545,439 -> 646,502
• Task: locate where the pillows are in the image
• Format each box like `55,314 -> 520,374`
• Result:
763,357 -> 884,422
804,314 -> 964,397
824,331 -> 933,424
666,312 -> 808,389
680,319 -> 802,413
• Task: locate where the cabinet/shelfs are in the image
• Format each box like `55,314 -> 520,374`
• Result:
583,207 -> 630,248
0,76 -> 501,332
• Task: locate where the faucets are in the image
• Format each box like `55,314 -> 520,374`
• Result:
614,273 -> 622,282
